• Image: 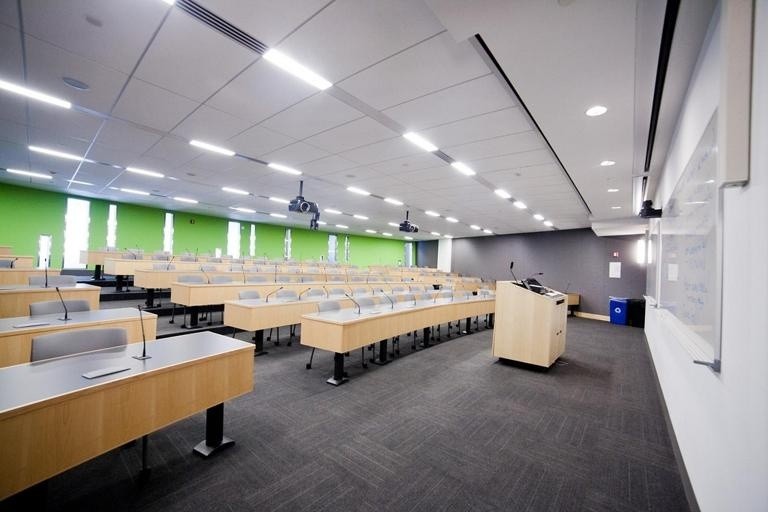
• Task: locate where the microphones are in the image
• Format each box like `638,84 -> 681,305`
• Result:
56,287 -> 71,320
367,271 -> 371,284
323,267 -> 327,283
409,286 -> 416,306
131,305 -> 153,360
274,266 -> 277,283
389,284 -> 393,294
299,287 -> 311,300
510,261 -> 517,283
345,293 -> 360,314
43,258 -> 50,288
451,286 -> 453,302
528,272 -> 544,286
344,270 -> 348,284
463,285 -> 469,299
167,255 -> 175,270
380,290 -> 393,309
200,268 -> 209,283
185,248 -> 191,257
433,286 -> 443,303
10,258 -> 18,268
368,285 -> 374,295
266,286 -> 283,302
195,248 -> 199,262
348,286 -> 353,297
208,250 -> 213,257
125,248 -> 136,260
136,244 -> 140,254
322,286 -> 328,299
243,253 -> 244,264
242,266 -> 246,284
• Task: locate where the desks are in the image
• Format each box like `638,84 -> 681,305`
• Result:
79,248 -> 497,388
0,252 -> 255,501
490,281 -> 569,368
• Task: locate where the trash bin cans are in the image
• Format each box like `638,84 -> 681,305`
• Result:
609,298 -> 645,328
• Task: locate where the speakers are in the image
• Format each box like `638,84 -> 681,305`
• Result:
627,299 -> 646,327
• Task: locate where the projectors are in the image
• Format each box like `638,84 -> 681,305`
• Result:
399,220 -> 419,232
289,197 -> 320,214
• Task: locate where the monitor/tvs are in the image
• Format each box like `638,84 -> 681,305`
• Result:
521,277 -> 545,294
